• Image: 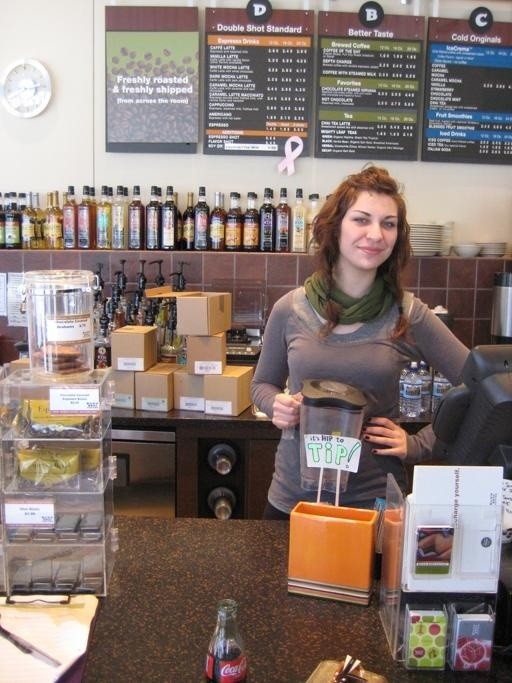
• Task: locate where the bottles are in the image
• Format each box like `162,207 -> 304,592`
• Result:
22,191 -> 68,250
63,185 -> 95,248
194,187 -> 210,250
306,193 -> 333,253
399,360 -> 454,419
211,191 -> 259,251
203,597 -> 249,683
96,186 -> 128,249
172,192 -> 195,249
259,187 -> 306,253
128,186 -> 176,250
0,192 -> 26,250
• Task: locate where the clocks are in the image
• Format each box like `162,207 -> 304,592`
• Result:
0,57 -> 51,118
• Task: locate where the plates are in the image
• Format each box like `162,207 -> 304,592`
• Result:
476,241 -> 509,258
408,223 -> 446,256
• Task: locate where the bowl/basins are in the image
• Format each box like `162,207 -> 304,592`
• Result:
451,244 -> 485,257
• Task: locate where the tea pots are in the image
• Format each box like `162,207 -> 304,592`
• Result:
294,377 -> 368,493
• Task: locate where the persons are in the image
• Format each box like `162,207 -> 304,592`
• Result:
249,164 -> 472,519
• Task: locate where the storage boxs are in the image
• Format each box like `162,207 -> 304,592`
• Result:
105,288 -> 254,417
